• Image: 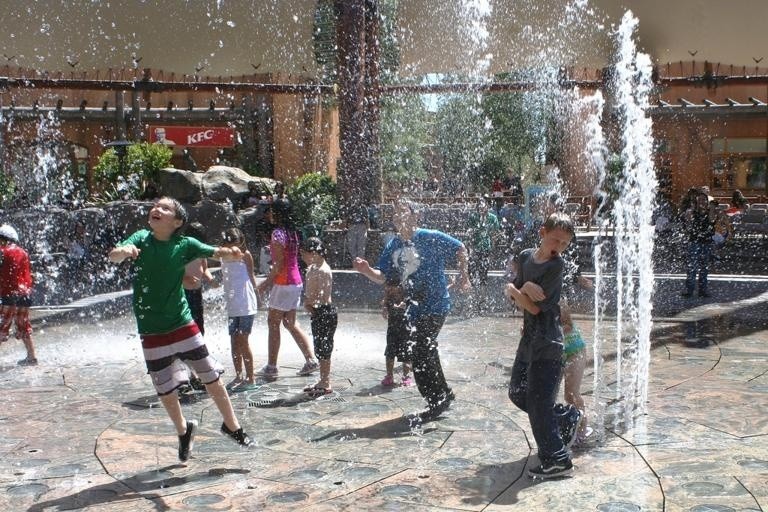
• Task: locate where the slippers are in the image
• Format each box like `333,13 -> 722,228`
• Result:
304,385 -> 333,398
570,427 -> 593,450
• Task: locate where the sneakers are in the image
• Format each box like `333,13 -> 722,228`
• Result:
529,457 -> 573,478
221,422 -> 251,446
421,391 -> 455,418
177,420 -> 197,461
18,356 -> 38,366
253,363 -> 277,376
557,408 -> 583,447
297,356 -> 320,375
226,377 -> 256,392
402,376 -> 411,386
190,376 -> 206,390
380,373 -> 394,385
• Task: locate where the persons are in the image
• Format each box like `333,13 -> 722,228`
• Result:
152,128 -> 176,146
257,202 -> 321,381
653,179 -> 749,296
108,196 -> 257,462
466,201 -> 577,289
340,199 -> 396,269
298,237 -> 339,394
352,199 -> 470,420
184,226 -> 221,391
206,228 -> 258,393
503,212 -> 594,479
139,182 -> 159,200
0,224 -> 38,366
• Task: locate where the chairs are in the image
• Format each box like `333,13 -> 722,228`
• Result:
377,197 -> 583,239
718,202 -> 768,233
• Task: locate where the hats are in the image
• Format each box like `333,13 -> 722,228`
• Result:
1,225 -> 18,243
